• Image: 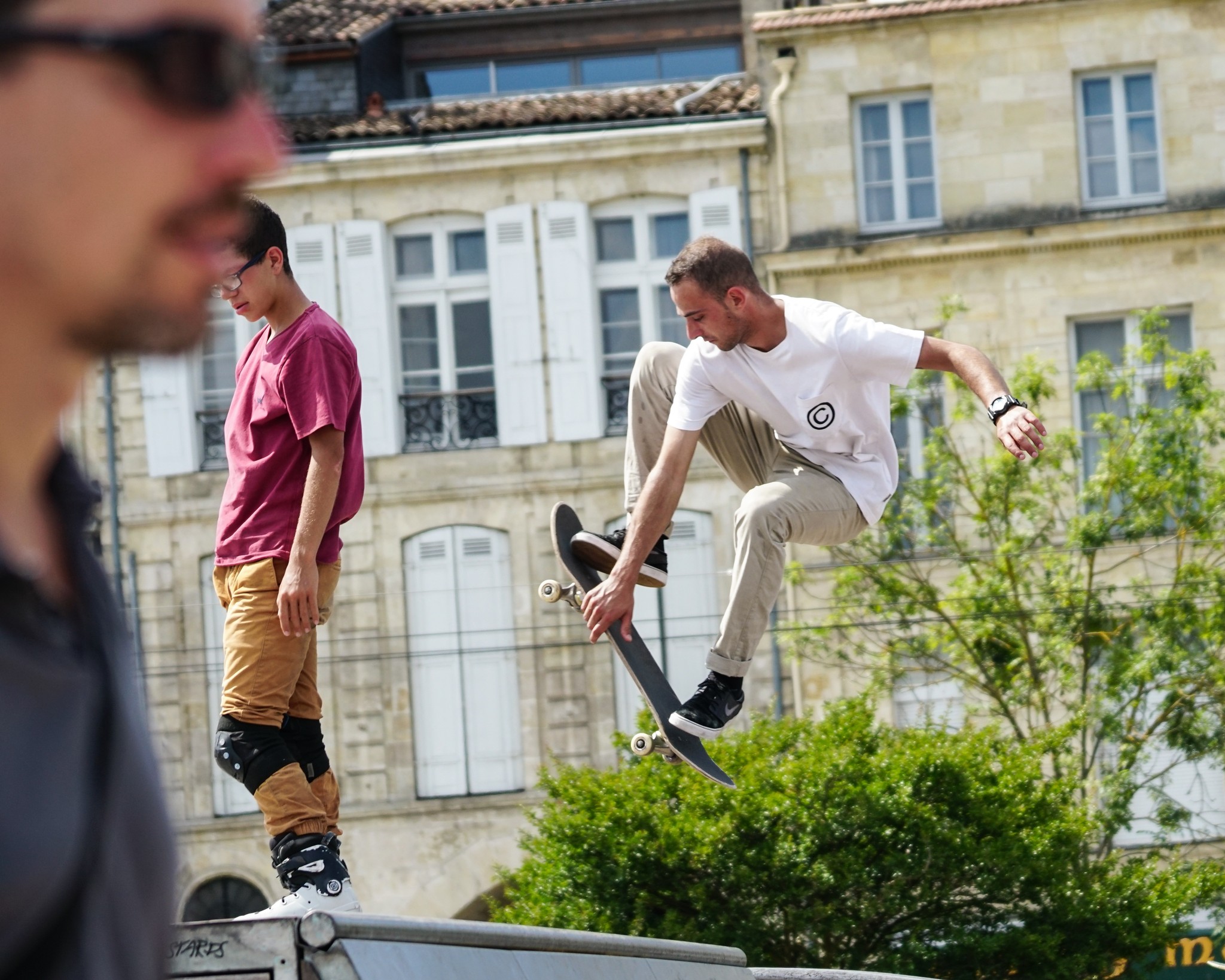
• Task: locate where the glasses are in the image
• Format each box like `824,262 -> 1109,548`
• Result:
209,250 -> 266,298
8,23 -> 279,112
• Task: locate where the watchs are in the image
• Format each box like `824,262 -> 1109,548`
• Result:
985,395 -> 1029,427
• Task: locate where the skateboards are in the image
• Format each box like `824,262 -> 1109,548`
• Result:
538,500 -> 736,789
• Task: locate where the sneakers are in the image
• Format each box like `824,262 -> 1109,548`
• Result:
231,877 -> 362,921
668,671 -> 745,738
570,529 -> 668,588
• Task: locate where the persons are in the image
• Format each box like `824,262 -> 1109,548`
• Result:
575,234 -> 1049,740
200,193 -> 372,924
0,4 -> 290,980
